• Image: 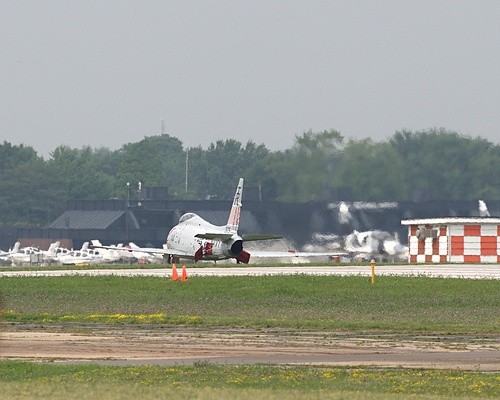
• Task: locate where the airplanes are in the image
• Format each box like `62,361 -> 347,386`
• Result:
90,177 -> 349,264
1,242 -> 166,264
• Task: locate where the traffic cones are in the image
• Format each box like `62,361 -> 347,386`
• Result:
170,262 -> 178,281
180,265 -> 188,282
